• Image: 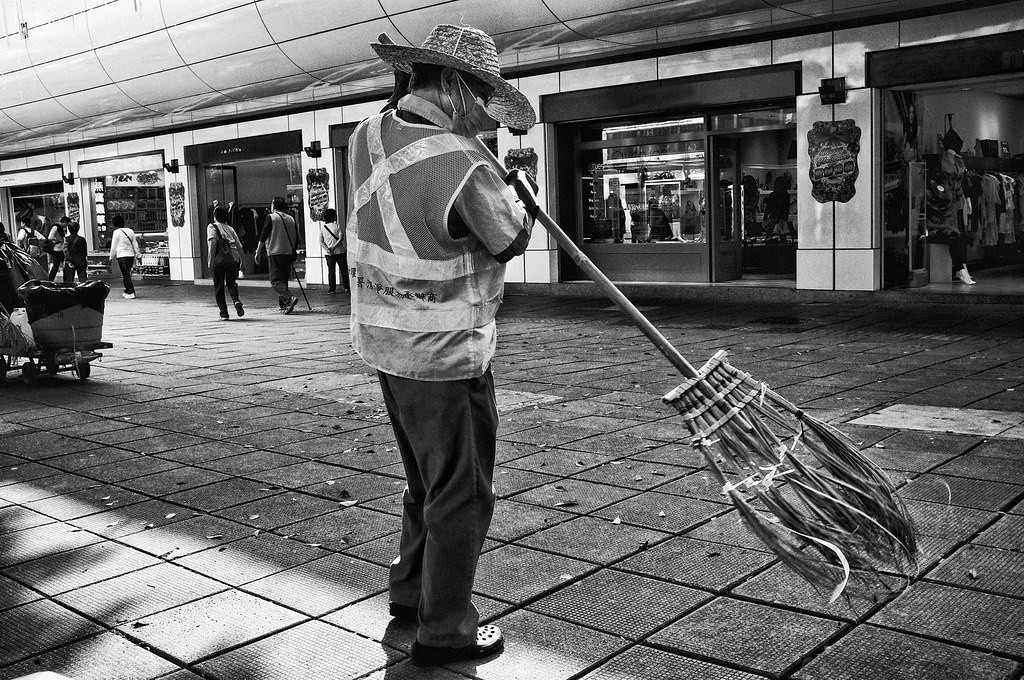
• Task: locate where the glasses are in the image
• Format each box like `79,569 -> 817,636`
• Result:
463,70 -> 496,107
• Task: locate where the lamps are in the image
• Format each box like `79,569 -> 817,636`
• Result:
303,141 -> 321,158
164,159 -> 178,173
62,173 -> 75,185
508,125 -> 528,137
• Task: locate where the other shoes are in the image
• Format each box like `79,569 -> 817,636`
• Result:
221,317 -> 229,320
278,307 -> 284,313
327,291 -> 335,294
235,301 -> 244,316
345,289 -> 350,292
122,293 -> 136,298
284,296 -> 298,314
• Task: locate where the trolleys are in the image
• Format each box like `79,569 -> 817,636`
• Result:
0,343 -> 114,388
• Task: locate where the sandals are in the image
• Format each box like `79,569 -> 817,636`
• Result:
410,624 -> 503,667
389,600 -> 418,621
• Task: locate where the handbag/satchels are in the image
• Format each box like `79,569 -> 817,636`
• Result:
211,225 -> 234,271
42,240 -> 54,253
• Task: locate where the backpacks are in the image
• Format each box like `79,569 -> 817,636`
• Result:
22,228 -> 41,258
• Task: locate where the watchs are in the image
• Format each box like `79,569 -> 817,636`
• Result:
685,200 -> 697,215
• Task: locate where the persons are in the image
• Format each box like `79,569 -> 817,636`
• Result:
0,222 -> 14,248
630,203 -> 645,243
659,186 -> 673,231
699,190 -> 706,241
18,219 -> 46,262
48,216 -> 88,282
648,193 -> 659,220
208,199 -> 224,224
346,24 -> 539,665
255,197 -> 299,315
319,208 -> 350,294
763,177 -> 790,243
742,175 -> 760,238
207,207 -> 244,320
109,215 -> 142,299
40,218 -> 51,238
924,185 -> 977,284
226,201 -> 244,278
903,104 -> 921,162
925,149 -> 973,281
606,191 -> 626,239
719,179 -> 732,238
646,204 -> 674,243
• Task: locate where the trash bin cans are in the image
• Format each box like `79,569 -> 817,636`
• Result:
17,280 -> 105,345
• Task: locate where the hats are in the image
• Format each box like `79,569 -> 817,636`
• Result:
369,23 -> 536,130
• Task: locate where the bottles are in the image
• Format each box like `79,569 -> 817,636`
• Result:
10,308 -> 34,346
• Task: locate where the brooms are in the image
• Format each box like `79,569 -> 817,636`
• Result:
0,302 -> 44,368
376,31 -> 921,622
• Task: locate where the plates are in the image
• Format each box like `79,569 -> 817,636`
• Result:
106,189 -> 135,211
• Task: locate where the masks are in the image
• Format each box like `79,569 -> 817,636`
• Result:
448,81 -> 491,138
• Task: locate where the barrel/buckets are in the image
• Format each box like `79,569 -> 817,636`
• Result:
18,282 -> 109,343
